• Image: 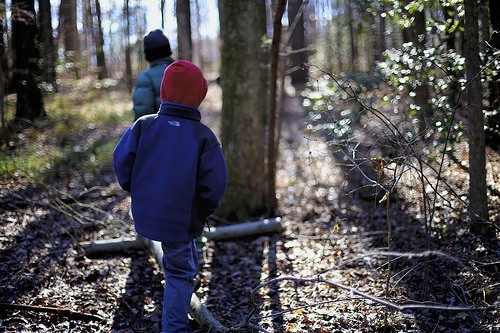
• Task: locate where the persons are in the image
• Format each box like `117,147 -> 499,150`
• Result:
111,59 -> 229,333
131,29 -> 176,121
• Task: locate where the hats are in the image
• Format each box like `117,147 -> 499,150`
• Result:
143,29 -> 173,61
